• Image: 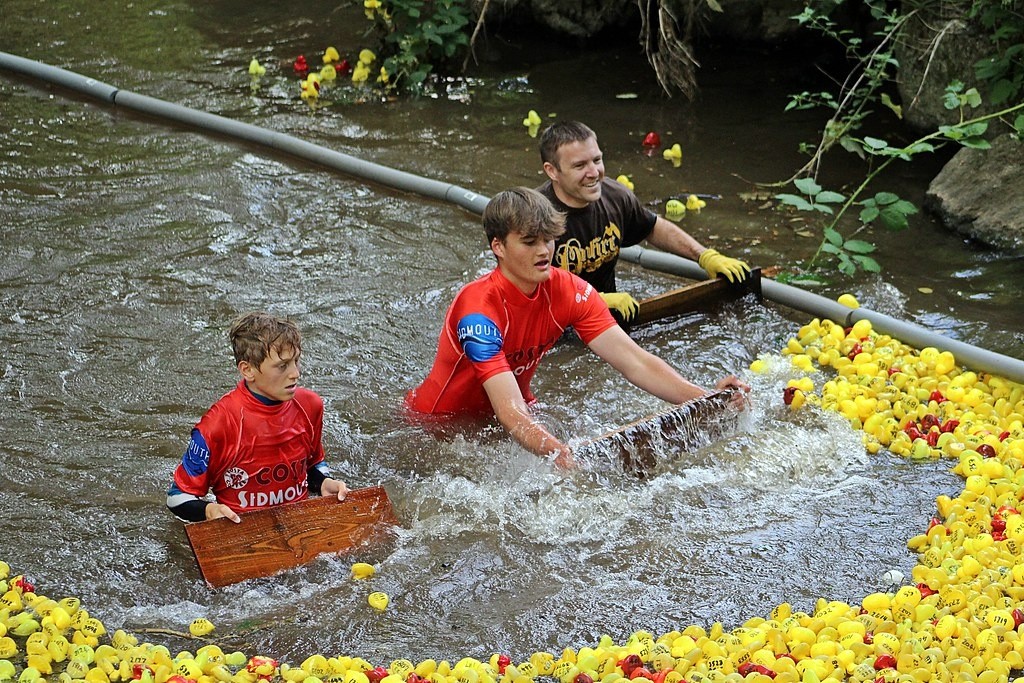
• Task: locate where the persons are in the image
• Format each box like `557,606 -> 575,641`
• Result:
166,312 -> 352,524
406,186 -> 751,470
532,119 -> 751,323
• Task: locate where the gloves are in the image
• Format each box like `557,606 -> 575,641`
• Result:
699,249 -> 753,285
599,292 -> 640,323
